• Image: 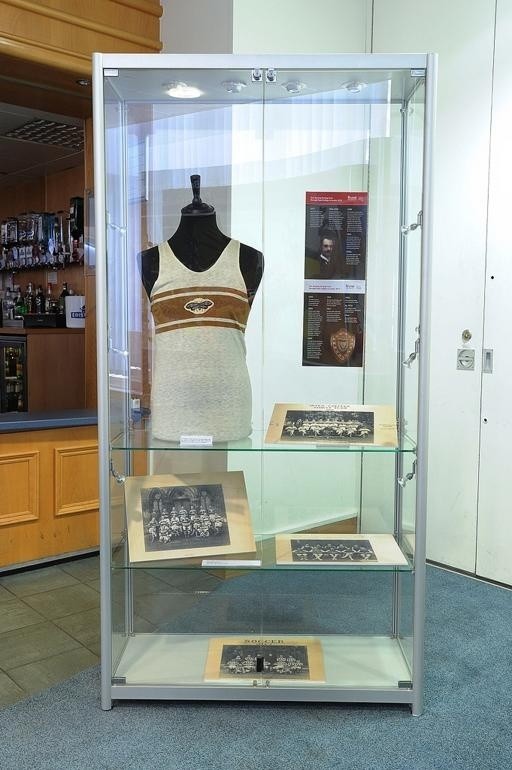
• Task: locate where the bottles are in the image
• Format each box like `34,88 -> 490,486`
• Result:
0,197 -> 84,272
2,345 -> 23,412
2,283 -> 82,320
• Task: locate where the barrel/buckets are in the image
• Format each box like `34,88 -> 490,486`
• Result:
65,296 -> 85,328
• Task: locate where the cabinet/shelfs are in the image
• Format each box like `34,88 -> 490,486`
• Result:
0,327 -> 86,413
91,50 -> 439,717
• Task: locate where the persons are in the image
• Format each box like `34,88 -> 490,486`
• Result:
292,542 -> 372,561
286,410 -> 374,438
142,503 -> 227,544
308,231 -> 338,278
142,200 -> 264,442
224,649 -> 307,675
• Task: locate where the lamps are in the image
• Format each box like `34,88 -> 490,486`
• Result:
160,82 -> 203,100
280,81 -> 306,95
337,80 -> 369,94
219,79 -> 246,94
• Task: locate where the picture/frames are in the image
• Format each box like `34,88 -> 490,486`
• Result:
205,635 -> 326,683
276,530 -> 409,566
121,471 -> 256,564
125,134 -> 149,204
263,403 -> 399,449
